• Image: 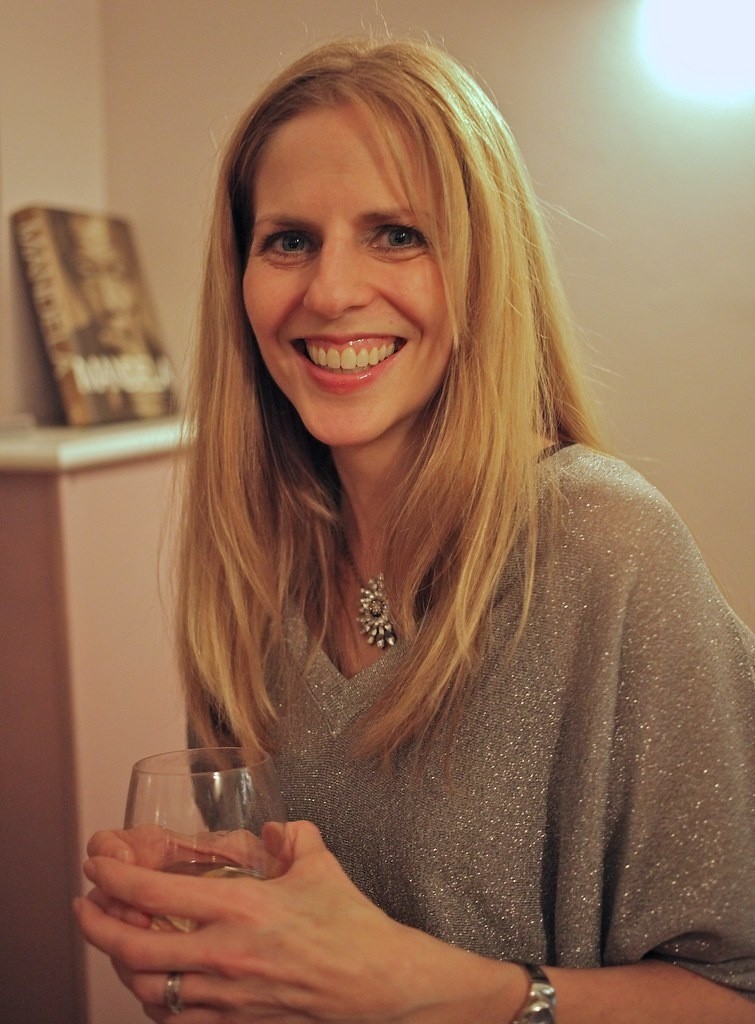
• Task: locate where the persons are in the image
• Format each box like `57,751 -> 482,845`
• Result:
54,211 -> 175,418
72,37 -> 754,1024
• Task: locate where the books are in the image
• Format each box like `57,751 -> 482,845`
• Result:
9,205 -> 179,427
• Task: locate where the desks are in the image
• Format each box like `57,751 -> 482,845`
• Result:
1,415 -> 210,1024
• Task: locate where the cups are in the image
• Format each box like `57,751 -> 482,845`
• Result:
123,747 -> 287,931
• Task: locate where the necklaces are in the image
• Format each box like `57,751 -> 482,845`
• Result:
341,540 -> 396,649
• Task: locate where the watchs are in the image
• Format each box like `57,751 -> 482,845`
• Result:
501,958 -> 556,1024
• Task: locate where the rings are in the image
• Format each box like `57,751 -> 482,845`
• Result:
166,973 -> 180,1012
